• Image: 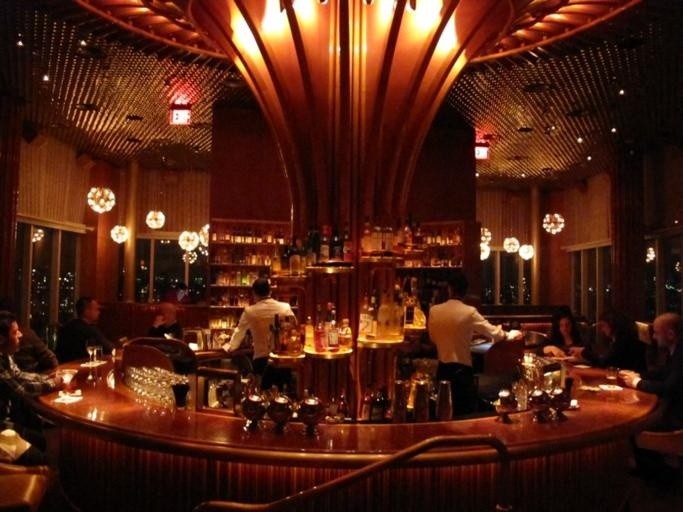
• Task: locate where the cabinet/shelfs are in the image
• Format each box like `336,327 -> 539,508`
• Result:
394,220 -> 465,324
209,218 -> 298,329
22,336 -> 667,512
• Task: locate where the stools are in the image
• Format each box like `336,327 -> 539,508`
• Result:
0,460 -> 60,512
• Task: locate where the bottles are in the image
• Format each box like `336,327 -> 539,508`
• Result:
360,391 -> 386,421
215,269 -> 252,286
327,309 -> 336,322
362,216 -> 370,257
383,225 -> 394,254
290,236 -> 300,274
314,304 -> 322,328
373,225 -> 381,255
315,324 -> 327,352
328,320 -> 340,352
271,312 -> 304,355
328,391 -> 350,419
397,222 -> 422,254
281,246 -> 290,276
333,231 -> 341,259
359,297 -> 368,336
389,292 -> 402,336
304,316 -> 314,350
343,231 -> 353,259
300,247 -> 307,272
319,230 -> 330,261
324,302 -> 334,347
377,288 -> 389,338
424,230 -> 462,246
213,225 -> 285,245
213,247 -> 280,267
365,295 -> 377,339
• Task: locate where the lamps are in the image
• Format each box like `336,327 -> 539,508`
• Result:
542,213 -> 565,235
87,158 -> 116,213
503,196 -> 519,254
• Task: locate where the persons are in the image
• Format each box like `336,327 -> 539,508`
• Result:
0,311 -> 63,429
588,310 -> 648,370
164,283 -> 191,304
146,302 -> 183,339
618,313 -> 682,432
222,278 -> 298,374
428,275 -> 506,417
56,297 -> 122,364
551,309 -> 584,354
15,329 -> 58,376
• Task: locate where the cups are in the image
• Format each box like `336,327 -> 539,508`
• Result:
58,370 -> 78,395
87,347 -> 94,365
94,347 -> 103,362
606,368 -> 619,388
127,365 -> 187,407
214,331 -> 224,350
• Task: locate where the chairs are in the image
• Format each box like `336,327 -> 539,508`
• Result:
475,336 -> 527,415
